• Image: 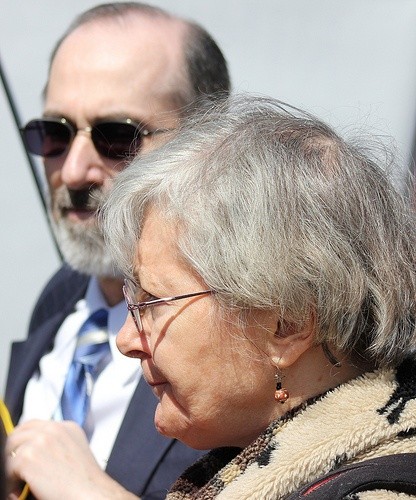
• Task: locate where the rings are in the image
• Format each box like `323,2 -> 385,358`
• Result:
12,449 -> 17,458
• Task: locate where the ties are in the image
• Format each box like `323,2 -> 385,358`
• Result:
51,309 -> 111,433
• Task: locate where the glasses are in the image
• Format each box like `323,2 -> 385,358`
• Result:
20,118 -> 195,161
122,277 -> 342,367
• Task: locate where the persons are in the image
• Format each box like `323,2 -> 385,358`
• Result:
0,2 -> 230,500
6,92 -> 416,500
